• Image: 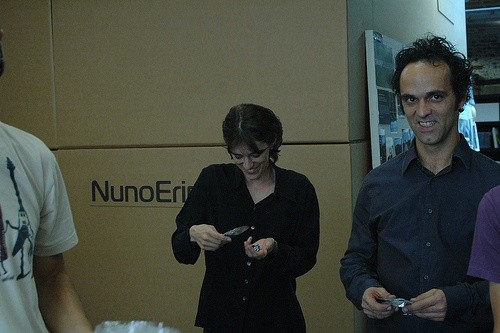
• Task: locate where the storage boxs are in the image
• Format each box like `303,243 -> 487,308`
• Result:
0,0 -> 480,332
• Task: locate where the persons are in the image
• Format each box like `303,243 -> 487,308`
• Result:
0,120 -> 93,333
339,35 -> 500,333
170,104 -> 320,333
